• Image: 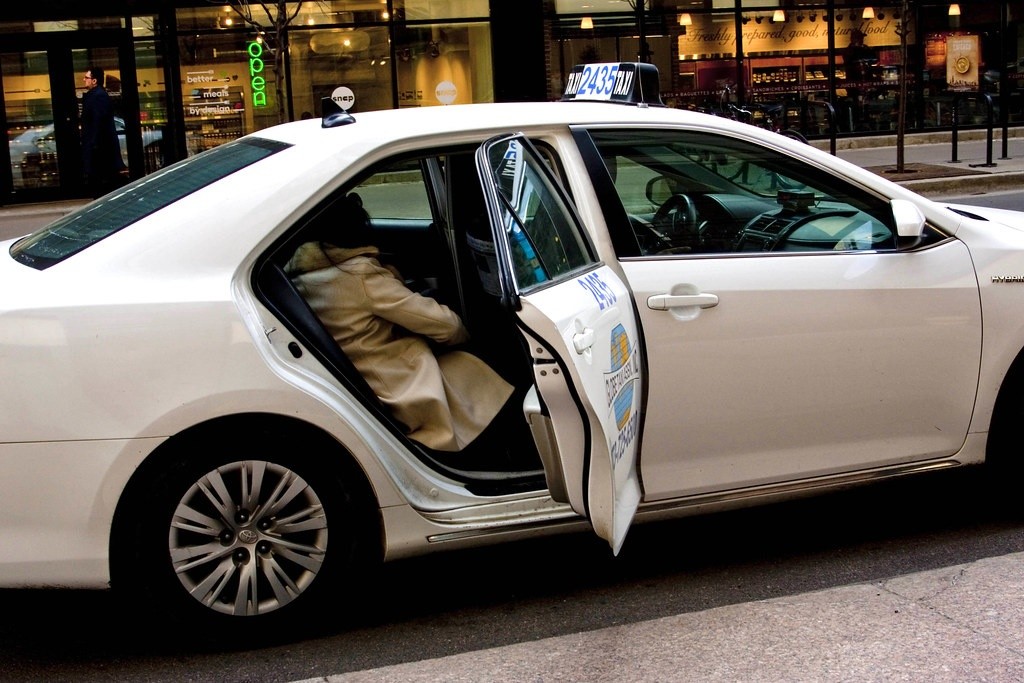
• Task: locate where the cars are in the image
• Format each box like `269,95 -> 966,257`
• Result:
8,115 -> 163,168
0,61 -> 1024,652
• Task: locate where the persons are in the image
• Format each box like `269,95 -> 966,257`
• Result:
76,65 -> 128,202
263,193 -> 545,474
841,27 -> 880,133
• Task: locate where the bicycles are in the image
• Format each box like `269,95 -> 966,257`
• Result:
711,80 -> 812,190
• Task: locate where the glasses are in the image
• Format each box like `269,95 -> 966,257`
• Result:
84,76 -> 92,80
